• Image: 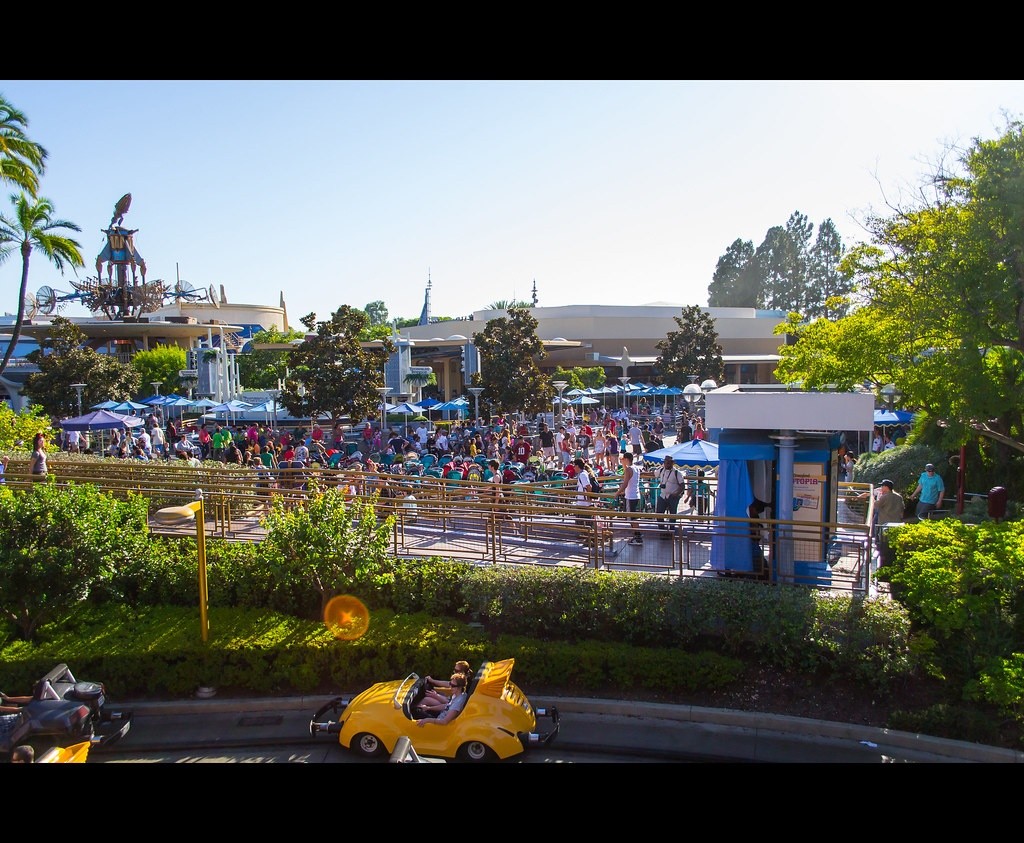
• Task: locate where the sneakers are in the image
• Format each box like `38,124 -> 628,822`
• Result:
628,538 -> 643,545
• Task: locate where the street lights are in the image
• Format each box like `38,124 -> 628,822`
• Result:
375,388 -> 393,428
70,382 -> 87,417
266,390 -> 282,429
682,383 -> 702,413
154,489 -> 217,699
617,377 -> 631,408
552,384 -> 569,426
468,388 -> 485,429
150,382 -> 163,396
880,382 -> 903,411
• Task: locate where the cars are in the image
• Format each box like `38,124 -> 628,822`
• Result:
339,657 -> 536,764
0,662 -> 107,763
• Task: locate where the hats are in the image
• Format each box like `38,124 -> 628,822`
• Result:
878,479 -> 894,489
661,456 -> 675,466
925,464 -> 934,471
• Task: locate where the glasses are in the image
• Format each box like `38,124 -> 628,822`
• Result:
454,668 -> 462,673
449,682 -> 458,687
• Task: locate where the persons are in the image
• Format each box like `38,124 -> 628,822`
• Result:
910,464 -> 945,524
0,456 -> 10,485
749,500 -> 769,572
29,433 -> 47,482
411,661 -> 473,727
0,681 -> 39,713
839,426 -> 911,482
61,405 -> 470,524
857,479 -> 905,539
11,746 -> 34,763
470,398 -> 717,549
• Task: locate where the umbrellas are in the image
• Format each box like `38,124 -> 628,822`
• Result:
87,393 -> 469,437
60,409 -> 145,457
484,381 -> 684,428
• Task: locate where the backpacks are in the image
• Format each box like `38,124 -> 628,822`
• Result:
579,471 -> 600,500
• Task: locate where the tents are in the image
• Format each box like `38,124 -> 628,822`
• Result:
643,437 -> 720,522
857,406 -> 917,455
